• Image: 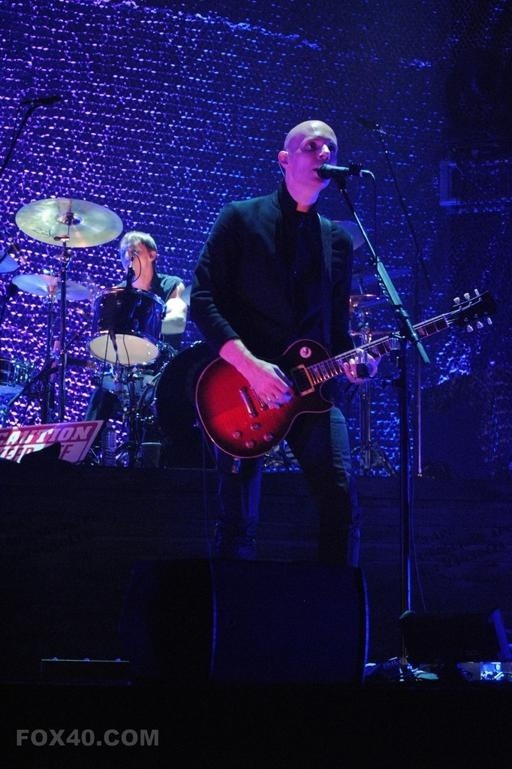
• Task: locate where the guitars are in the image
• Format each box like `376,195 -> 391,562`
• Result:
196,288 -> 495,458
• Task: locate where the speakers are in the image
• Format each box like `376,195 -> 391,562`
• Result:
154,556 -> 368,685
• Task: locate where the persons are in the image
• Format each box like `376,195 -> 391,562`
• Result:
191,120 -> 377,566
92,232 -> 191,352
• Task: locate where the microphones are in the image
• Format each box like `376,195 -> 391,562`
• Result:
317,163 -> 376,183
36,96 -> 60,105
125,255 -> 135,280
358,115 -> 378,129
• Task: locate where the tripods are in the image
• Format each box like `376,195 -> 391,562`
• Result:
368,326 -> 451,683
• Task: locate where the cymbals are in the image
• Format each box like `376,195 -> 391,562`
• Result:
17,198 -> 123,248
12,274 -> 92,302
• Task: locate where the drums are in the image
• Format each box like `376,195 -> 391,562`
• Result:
86,286 -> 168,367
94,344 -> 180,398
137,339 -> 259,473
